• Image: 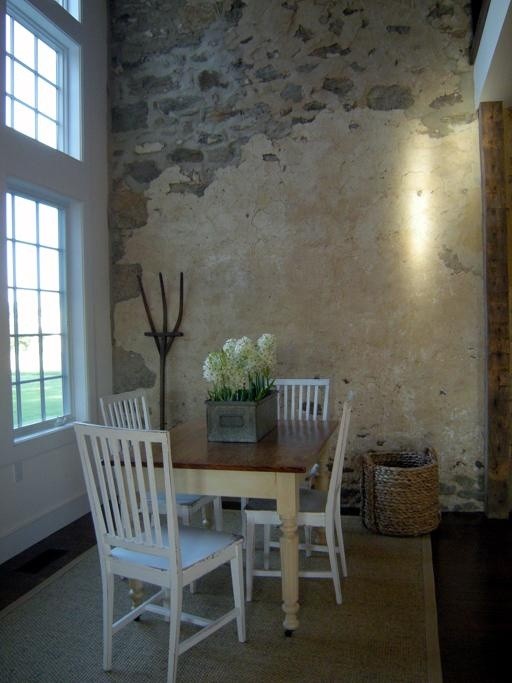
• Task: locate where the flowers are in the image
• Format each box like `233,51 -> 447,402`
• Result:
199,332 -> 281,401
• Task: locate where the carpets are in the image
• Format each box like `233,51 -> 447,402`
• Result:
1,513 -> 444,681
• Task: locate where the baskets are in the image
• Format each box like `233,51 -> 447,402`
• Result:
360,446 -> 442,537
